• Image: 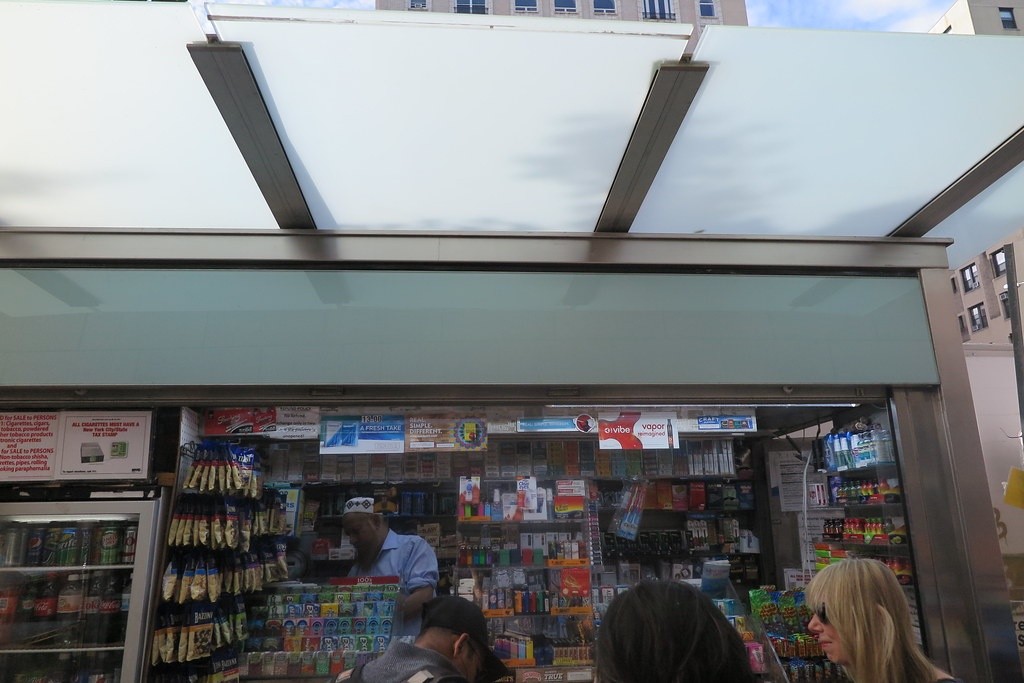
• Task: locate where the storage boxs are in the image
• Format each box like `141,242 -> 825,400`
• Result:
502,505 -> 518,521
458,516 -> 491,521
592,587 -> 599,604
499,657 -> 535,668
616,587 -> 629,596
238,586 -> 398,676
551,606 -> 593,614
482,607 -> 515,617
564,543 -> 572,559
513,667 -> 593,683
523,487 -> 547,520
722,484 -> 738,509
672,484 -> 688,511
546,532 -> 558,557
554,478 -> 586,496
548,558 -> 589,566
616,561 -> 640,586
688,482 -> 705,510
736,482 -> 755,510
554,495 -> 584,519
671,563 -> 693,580
532,533 -> 546,558
416,523 -> 456,559
572,543 -> 578,558
280,487 -> 306,539
640,482 -> 656,509
458,475 -> 480,504
558,532 -> 571,542
501,493 -> 518,505
706,481 -> 723,509
656,481 -> 672,510
311,527 -> 358,560
744,641 -> 765,672
599,586 -> 615,603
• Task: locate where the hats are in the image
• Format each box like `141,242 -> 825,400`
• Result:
421,596 -> 508,683
343,497 -> 386,515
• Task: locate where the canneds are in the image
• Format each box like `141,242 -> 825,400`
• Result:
0,525 -> 139,568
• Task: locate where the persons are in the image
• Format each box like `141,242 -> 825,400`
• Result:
325,595 -> 508,683
591,579 -> 754,683
339,497 -> 438,641
806,558 -> 960,683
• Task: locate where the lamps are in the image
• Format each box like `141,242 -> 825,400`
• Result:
812,419 -> 824,471
734,436 -> 752,466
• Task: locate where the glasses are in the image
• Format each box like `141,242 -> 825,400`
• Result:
816,606 -> 830,625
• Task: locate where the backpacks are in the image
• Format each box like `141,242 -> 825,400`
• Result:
330,663 -> 468,683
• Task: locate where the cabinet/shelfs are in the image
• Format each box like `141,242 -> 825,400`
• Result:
238,575 -> 403,679
455,463 -> 925,683
289,476 -> 455,565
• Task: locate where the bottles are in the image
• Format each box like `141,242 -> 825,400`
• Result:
864,555 -> 911,583
460,545 -> 492,565
834,430 -> 894,470
823,518 -> 895,534
571,591 -> 582,607
473,584 -> 550,613
495,637 -> 533,659
458,500 -> 491,517
836,478 -> 889,504
1,571 -> 135,683
